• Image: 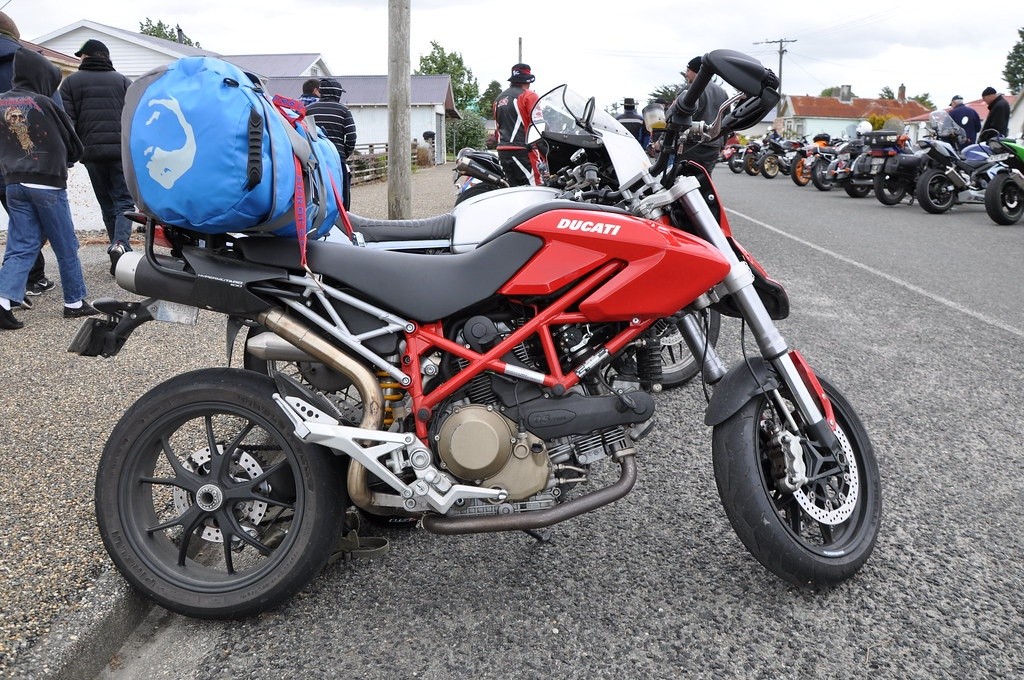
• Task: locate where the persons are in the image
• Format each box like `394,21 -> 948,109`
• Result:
673,56 -> 731,177
0,46 -> 101,330
305,78 -> 357,211
0,11 -> 74,310
978,87 -> 1010,137
298,79 -> 321,108
942,95 -> 981,144
642,98 -> 666,137
59,39 -> 133,276
615,98 -> 648,150
492,63 -> 550,187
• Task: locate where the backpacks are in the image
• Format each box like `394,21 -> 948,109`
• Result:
122,54 -> 350,242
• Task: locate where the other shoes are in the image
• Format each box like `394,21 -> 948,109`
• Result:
110,241 -> 126,276
62,299 -> 100,318
0,304 -> 24,330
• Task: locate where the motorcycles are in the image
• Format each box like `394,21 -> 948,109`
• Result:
719,110 -> 1022,227
67,51 -> 884,621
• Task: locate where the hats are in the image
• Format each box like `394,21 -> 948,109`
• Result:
948,95 -> 964,106
74,39 -> 109,57
686,56 -> 704,74
507,63 -> 535,83
620,98 -> 639,106
981,87 -> 997,97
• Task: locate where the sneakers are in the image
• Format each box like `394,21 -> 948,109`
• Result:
23,285 -> 42,296
39,280 -> 56,292
19,296 -> 34,309
